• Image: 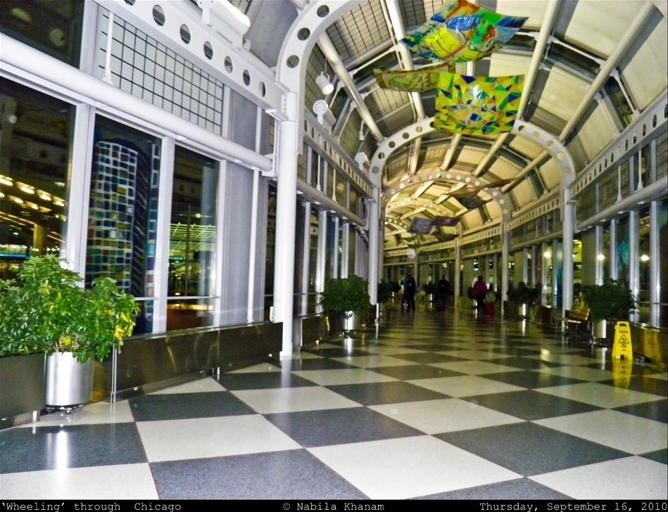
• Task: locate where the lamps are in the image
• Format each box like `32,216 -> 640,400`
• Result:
315,61 -> 335,95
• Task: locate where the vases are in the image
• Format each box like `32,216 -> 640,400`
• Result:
43,350 -> 93,414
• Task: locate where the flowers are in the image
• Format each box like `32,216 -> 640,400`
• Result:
0,256 -> 139,364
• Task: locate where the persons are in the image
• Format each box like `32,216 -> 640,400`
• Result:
473,275 -> 487,314
438,274 -> 450,311
404,272 -> 417,312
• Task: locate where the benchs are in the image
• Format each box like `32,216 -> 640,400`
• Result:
568,307 -> 591,332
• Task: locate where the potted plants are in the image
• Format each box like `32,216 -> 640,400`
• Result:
317,274 -> 372,333
506,281 -> 543,319
580,277 -> 637,341
